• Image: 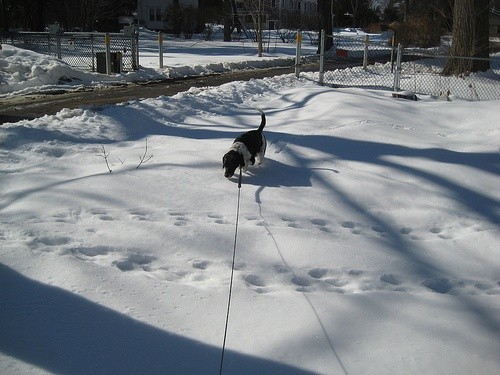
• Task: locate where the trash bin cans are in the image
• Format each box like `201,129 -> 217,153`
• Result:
96,51 -> 123,74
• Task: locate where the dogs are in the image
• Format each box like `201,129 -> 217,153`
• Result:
222,108 -> 268,178
